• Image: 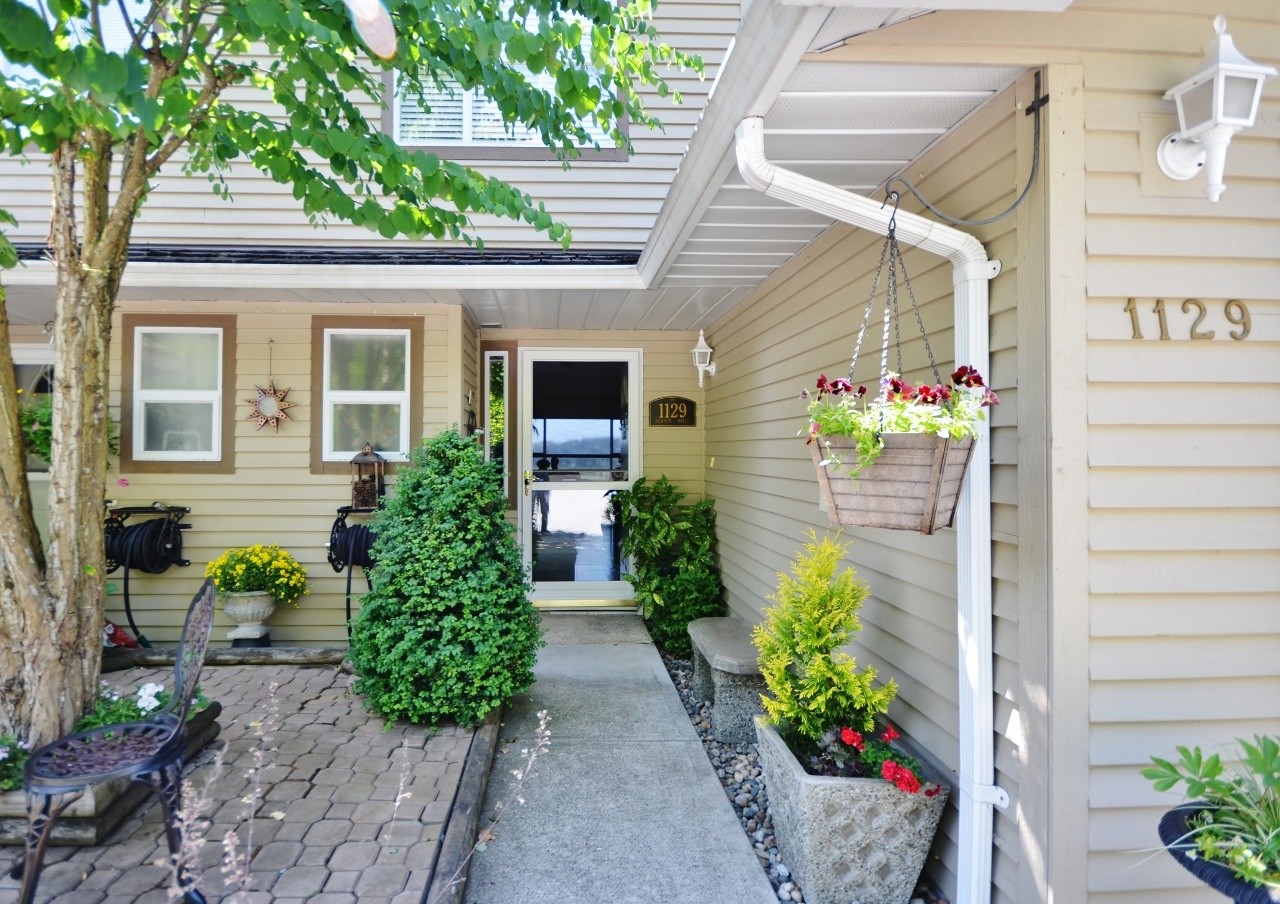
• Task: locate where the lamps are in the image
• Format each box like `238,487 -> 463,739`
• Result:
1157,14 -> 1280,208
689,329 -> 716,387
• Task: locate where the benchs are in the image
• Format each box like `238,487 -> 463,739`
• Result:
685,616 -> 774,745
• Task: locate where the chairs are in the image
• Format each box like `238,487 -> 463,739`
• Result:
8,574 -> 219,903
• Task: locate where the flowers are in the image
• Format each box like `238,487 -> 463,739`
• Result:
799,359 -> 999,479
18,395 -> 131,489
837,721 -> 944,798
204,542 -> 315,611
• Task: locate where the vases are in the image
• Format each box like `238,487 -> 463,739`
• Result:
218,588 -> 277,640
808,430 -> 980,536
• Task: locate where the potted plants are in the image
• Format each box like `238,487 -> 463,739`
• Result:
1141,734 -> 1279,904
739,531 -> 951,904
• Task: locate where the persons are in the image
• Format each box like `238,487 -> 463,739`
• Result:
531,472 -> 552,538
536,458 -> 551,470
550,456 -> 560,471
